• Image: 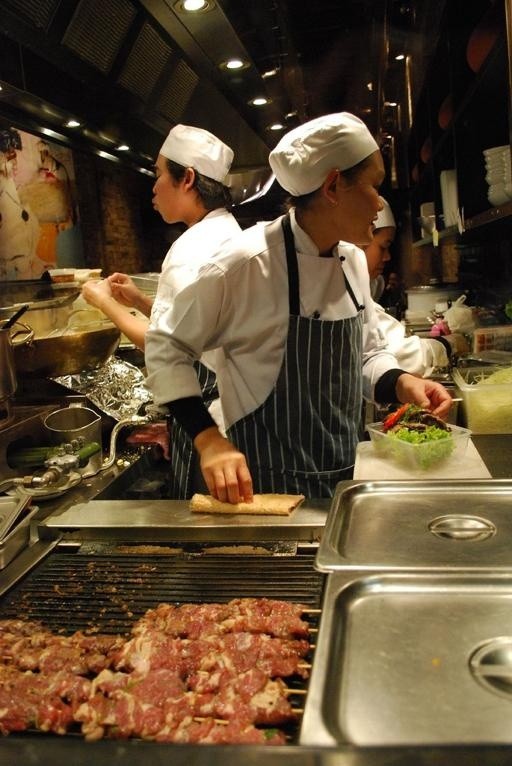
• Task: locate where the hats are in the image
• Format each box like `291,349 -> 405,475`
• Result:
268,112 -> 381,198
159,123 -> 237,183
374,195 -> 397,229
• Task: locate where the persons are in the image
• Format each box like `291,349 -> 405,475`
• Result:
146,111 -> 457,503
84,121 -> 250,501
357,191 -> 468,376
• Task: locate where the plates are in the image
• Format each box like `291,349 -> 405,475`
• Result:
481,145 -> 512,199
5,473 -> 83,500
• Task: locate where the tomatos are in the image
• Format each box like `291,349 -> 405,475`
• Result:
383,402 -> 409,430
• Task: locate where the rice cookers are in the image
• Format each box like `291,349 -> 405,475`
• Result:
404,278 -> 467,315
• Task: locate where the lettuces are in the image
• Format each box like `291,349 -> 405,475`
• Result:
373,425 -> 454,469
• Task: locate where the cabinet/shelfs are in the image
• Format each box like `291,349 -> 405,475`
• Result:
403,1 -> 512,250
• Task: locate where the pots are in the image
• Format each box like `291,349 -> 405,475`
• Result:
1,305 -> 129,388
